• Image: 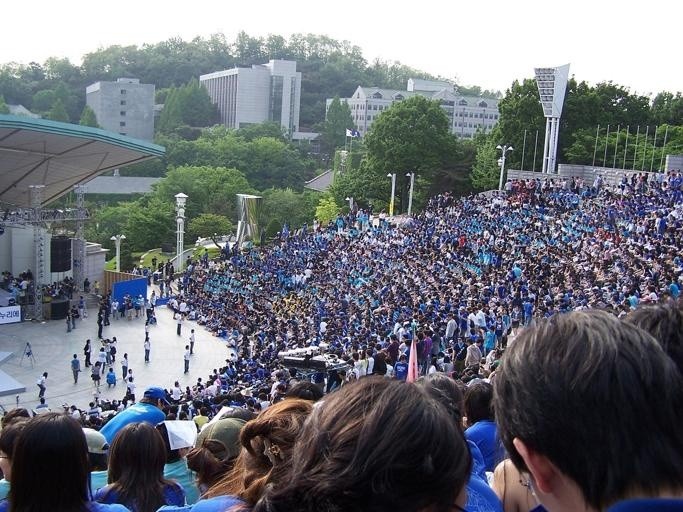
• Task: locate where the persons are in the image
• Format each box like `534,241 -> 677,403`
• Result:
1,168 -> 682,510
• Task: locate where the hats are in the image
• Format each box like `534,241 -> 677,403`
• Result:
196,417 -> 249,465
143,386 -> 173,407
83,427 -> 111,454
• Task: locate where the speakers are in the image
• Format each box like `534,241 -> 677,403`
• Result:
50,237 -> 71,274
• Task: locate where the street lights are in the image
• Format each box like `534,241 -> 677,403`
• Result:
406,171 -> 414,218
109,233 -> 126,271
385,172 -> 397,218
173,191 -> 188,272
344,196 -> 354,214
495,144 -> 513,193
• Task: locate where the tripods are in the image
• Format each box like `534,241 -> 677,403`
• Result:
19,342 -> 37,368
